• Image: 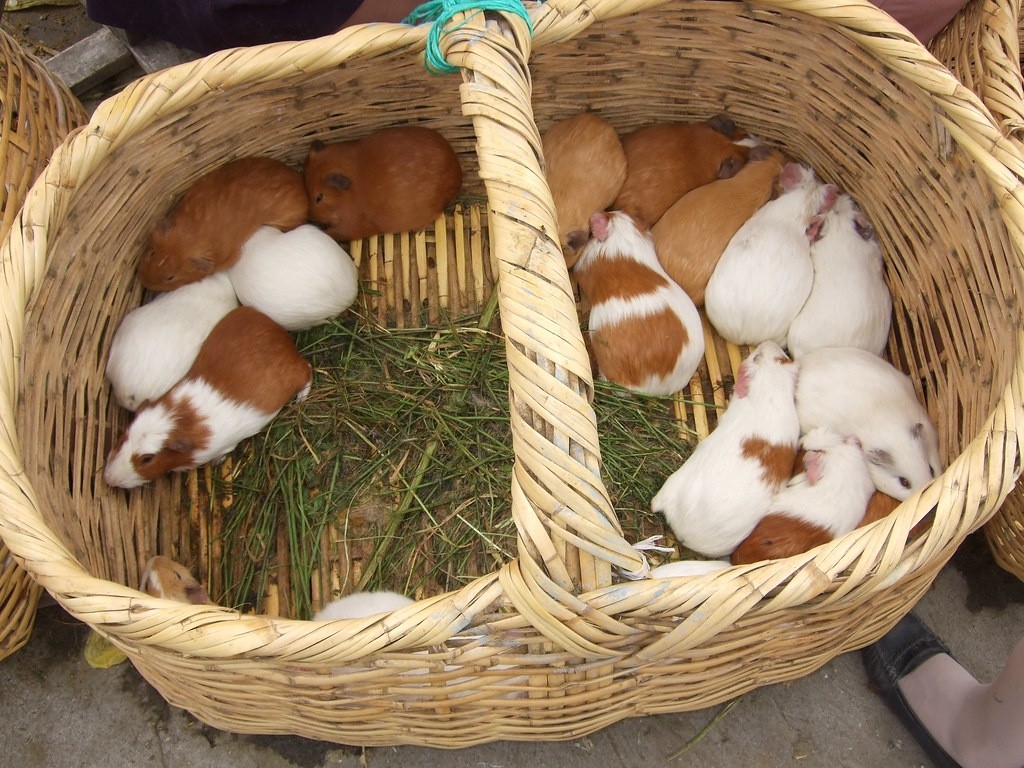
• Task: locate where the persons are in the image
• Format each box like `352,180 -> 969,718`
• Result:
860,608 -> 1023,768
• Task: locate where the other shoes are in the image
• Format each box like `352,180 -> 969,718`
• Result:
862,608 -> 981,768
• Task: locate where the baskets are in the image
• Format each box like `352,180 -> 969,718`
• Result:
0,0 -> 1024,749
